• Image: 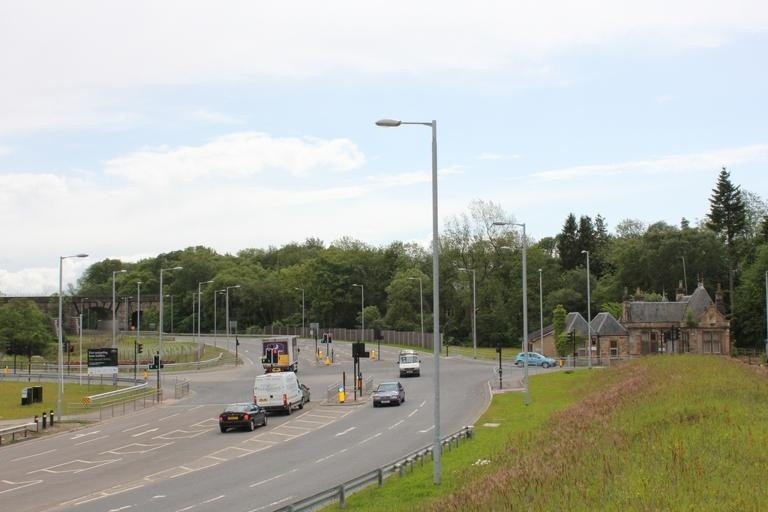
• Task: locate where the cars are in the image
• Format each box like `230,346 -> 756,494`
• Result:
515,352 -> 556,368
218,402 -> 267,430
299,383 -> 310,403
372,381 -> 405,406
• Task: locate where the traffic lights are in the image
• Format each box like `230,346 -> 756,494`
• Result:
323,334 -> 327,343
329,337 -> 331,343
62,340 -> 67,351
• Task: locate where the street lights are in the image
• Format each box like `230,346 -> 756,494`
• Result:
56,253 -> 90,419
494,219 -> 530,406
538,268 -> 544,356
111,265 -> 240,387
406,276 -> 425,348
580,250 -> 591,367
376,118 -> 442,486
294,287 -> 304,338
352,283 -> 364,342
79,296 -> 88,387
458,267 -> 478,359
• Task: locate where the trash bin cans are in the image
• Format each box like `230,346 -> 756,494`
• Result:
33,387 -> 42,402
22,387 -> 33,405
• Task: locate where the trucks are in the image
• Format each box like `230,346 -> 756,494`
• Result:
261,336 -> 299,372
397,349 -> 421,379
255,372 -> 304,414
